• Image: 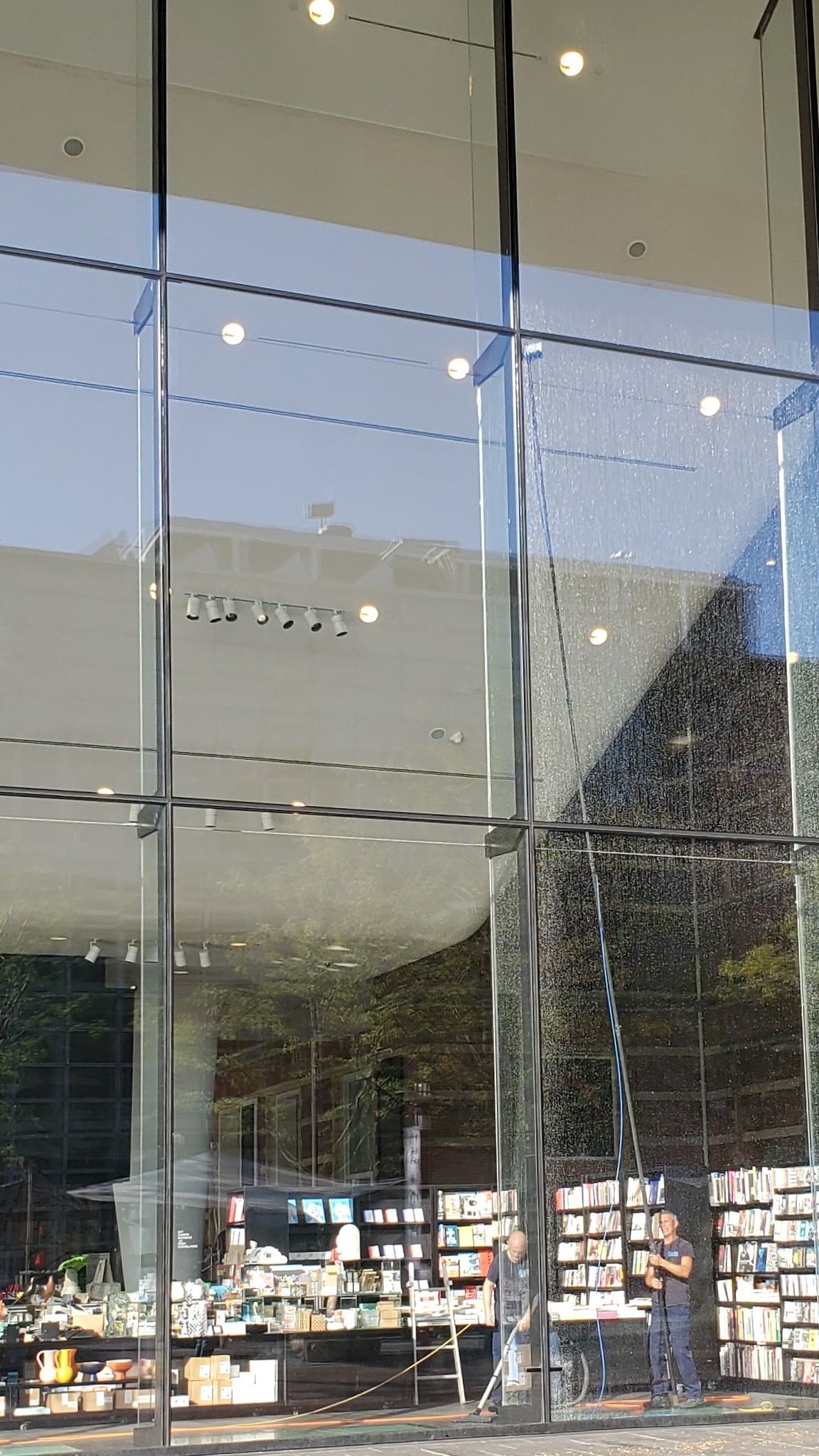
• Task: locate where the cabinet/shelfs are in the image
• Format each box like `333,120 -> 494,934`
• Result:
0,1377 -> 138,1431
226,1163 -> 818,1399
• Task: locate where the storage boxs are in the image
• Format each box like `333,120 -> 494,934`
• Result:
14,1355 -> 278,1418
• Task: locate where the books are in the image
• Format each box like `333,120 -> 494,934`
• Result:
717,1241 -> 777,1275
547,1290 -> 652,1323
560,1262 -> 624,1288
438,1216 -> 517,1248
714,1275 -> 780,1304
555,1175 -> 665,1211
715,1305 -> 781,1342
558,1237 -> 648,1274
435,1185 -> 517,1222
559,1210 -> 668,1239
718,1341 -> 783,1381
772,1165 -> 819,1384
705,1165 -> 772,1207
715,1208 -> 774,1238
439,1249 -> 494,1277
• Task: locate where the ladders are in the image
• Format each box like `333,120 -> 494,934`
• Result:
409,1260 -> 467,1404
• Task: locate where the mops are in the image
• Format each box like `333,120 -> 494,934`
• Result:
452,1327 -> 519,1423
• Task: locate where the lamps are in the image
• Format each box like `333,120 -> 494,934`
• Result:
250,600 -> 269,625
84,938 -> 101,964
331,610 -> 349,636
303,607 -> 323,632
174,940 -> 188,967
124,939 -> 139,965
199,940 -> 211,968
222,597 -> 238,622
274,603 -> 294,629
185,593 -> 202,620
261,811 -> 275,832
204,595 -> 222,623
205,807 -> 217,829
128,803 -> 141,822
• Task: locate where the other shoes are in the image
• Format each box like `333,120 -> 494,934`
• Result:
488,1399 -> 502,1412
643,1394 -> 671,1409
676,1395 -> 705,1408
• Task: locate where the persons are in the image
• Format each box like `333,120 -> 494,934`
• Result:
481,1231 -> 533,1409
643,1210 -> 703,1410
